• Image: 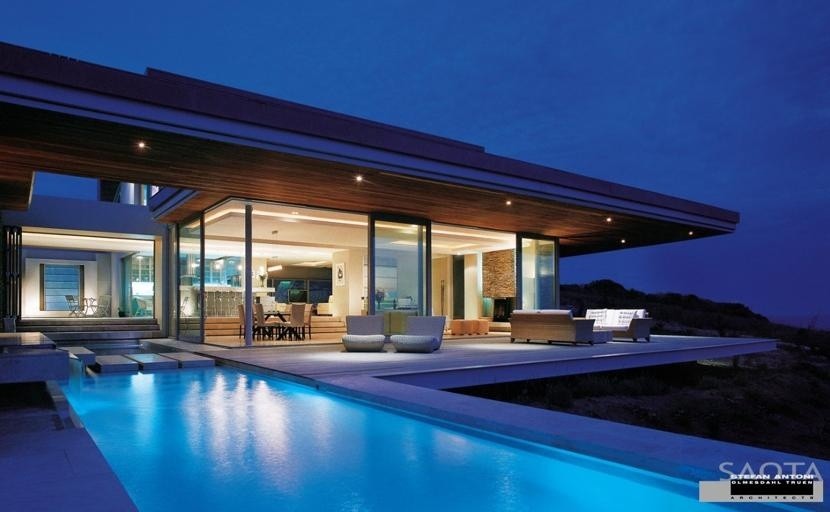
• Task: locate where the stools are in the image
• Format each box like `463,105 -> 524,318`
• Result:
449,318 -> 490,337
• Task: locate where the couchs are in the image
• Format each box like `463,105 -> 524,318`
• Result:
509,308 -> 652,345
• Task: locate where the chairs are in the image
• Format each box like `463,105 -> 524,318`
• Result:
341,313 -> 445,353
237,303 -> 313,341
65,294 -> 112,318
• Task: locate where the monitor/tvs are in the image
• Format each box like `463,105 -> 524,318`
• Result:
288,289 -> 310,303
